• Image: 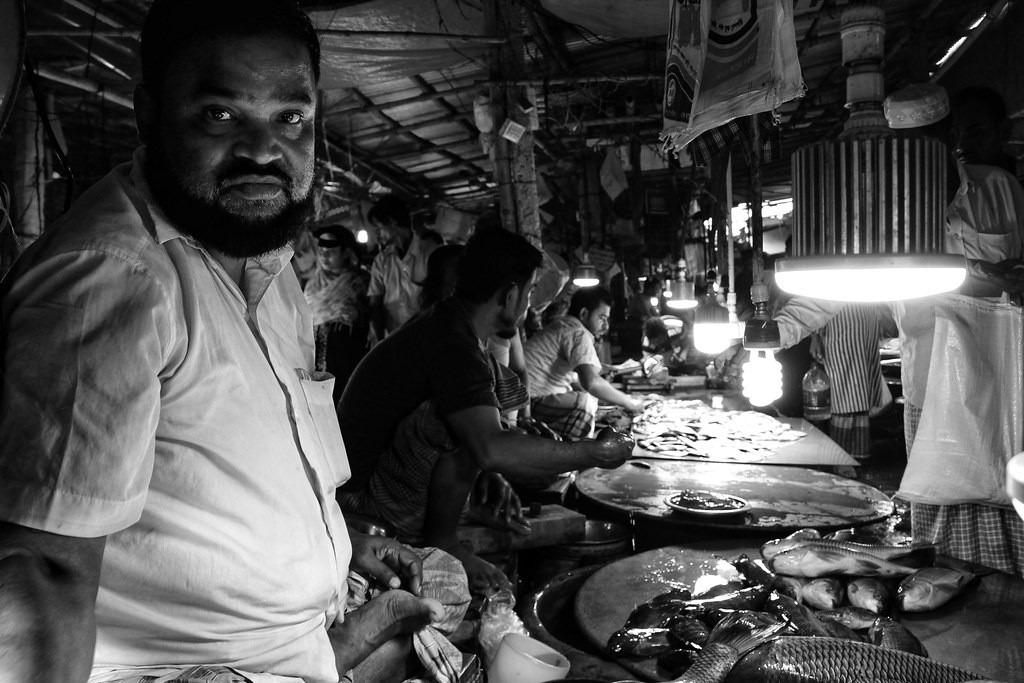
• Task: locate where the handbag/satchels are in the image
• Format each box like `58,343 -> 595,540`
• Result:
896,289 -> 1024,510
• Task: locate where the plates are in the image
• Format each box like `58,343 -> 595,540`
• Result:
664,493 -> 752,515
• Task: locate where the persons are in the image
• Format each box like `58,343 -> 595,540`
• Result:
1,1 -> 1024,683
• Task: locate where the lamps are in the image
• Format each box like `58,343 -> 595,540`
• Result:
772,111 -> 969,305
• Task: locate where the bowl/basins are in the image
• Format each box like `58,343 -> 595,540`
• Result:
565,519 -> 628,563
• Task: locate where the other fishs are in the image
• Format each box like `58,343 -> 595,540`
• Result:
608,527 -> 984,683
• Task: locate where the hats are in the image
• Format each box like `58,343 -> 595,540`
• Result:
314,224 -> 359,252
883,84 -> 950,129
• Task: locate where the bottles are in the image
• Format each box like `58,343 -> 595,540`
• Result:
803,363 -> 832,420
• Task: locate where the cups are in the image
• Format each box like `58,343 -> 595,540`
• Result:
487,633 -> 570,683
